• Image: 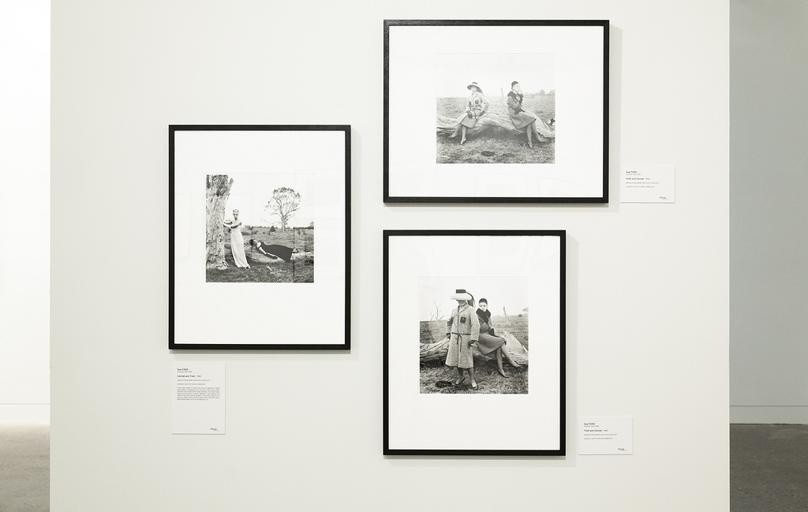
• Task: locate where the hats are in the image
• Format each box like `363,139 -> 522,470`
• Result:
467,81 -> 481,91
449,289 -> 473,301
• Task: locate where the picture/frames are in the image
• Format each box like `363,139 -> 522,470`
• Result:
167,124 -> 352,350
382,227 -> 568,456
383,18 -> 609,205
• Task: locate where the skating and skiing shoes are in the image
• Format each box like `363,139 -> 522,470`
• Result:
515,363 -> 527,370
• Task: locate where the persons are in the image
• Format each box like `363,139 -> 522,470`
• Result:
445,288 -> 480,390
222,209 -> 251,268
476,299 -> 525,377
447,82 -> 485,145
249,239 -> 299,262
507,81 -> 546,149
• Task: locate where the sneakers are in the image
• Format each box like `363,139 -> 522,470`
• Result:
471,380 -> 478,389
448,133 -> 466,144
456,376 -> 465,385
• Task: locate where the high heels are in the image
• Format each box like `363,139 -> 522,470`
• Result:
496,369 -> 509,378
